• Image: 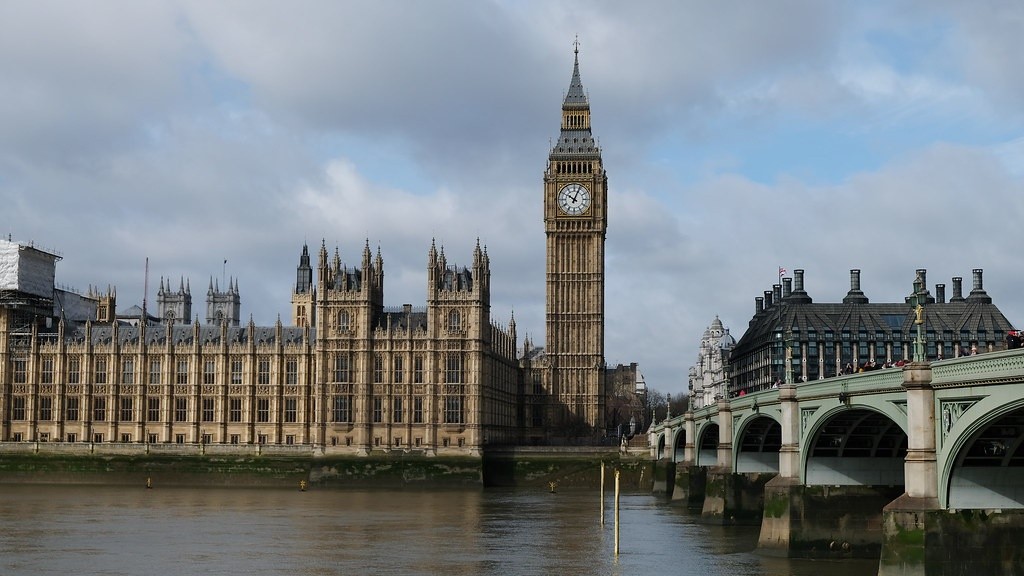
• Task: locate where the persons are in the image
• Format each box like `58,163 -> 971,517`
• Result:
736,329 -> 1023,397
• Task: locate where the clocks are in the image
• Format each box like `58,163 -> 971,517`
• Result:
557,181 -> 591,216
